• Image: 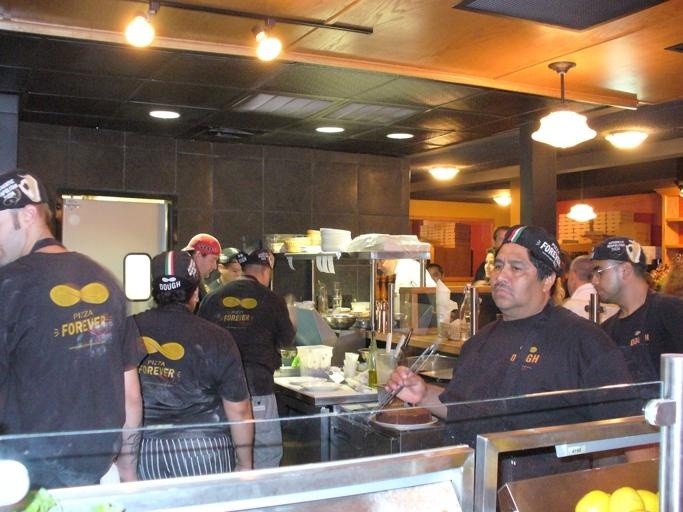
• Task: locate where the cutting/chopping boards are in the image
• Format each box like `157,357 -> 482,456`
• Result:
275,375 -> 378,405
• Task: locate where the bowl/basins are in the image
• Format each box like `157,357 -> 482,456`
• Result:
408,355 -> 458,381
266,228 -> 351,253
323,315 -> 355,329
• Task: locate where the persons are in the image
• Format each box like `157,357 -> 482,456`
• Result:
194,248 -> 298,472
205,245 -> 246,296
174,231 -> 222,318
378,222 -> 661,452
0,165 -> 148,492
586,236 -> 683,416
128,246 -> 257,484
396,225 -> 621,330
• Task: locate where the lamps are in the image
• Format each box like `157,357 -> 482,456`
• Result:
530,61 -> 597,149
121,0 -> 374,62
564,171 -> 598,222
429,168 -> 460,181
493,196 -> 512,207
604,128 -> 650,150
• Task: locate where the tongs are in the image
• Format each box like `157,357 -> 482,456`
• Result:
371,344 -> 440,416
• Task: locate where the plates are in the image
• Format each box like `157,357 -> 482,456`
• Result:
300,382 -> 341,392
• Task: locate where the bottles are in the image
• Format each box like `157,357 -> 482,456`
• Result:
317,284 -> 329,313
459,285 -> 480,342
368,331 -> 377,386
332,281 -> 343,309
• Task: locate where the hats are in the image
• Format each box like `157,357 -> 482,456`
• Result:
0,170 -> 48,211
503,225 -> 560,274
591,238 -> 644,268
151,233 -> 274,286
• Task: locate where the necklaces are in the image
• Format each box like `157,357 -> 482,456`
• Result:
240,273 -> 259,284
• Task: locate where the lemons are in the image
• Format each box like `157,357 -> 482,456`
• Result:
574,485 -> 609,512
608,486 -> 645,512
637,488 -> 660,512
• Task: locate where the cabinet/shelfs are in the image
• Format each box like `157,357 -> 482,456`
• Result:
654,185 -> 683,265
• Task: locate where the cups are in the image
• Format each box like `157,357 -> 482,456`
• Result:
378,357 -> 399,385
437,310 -> 451,339
342,352 -> 359,375
